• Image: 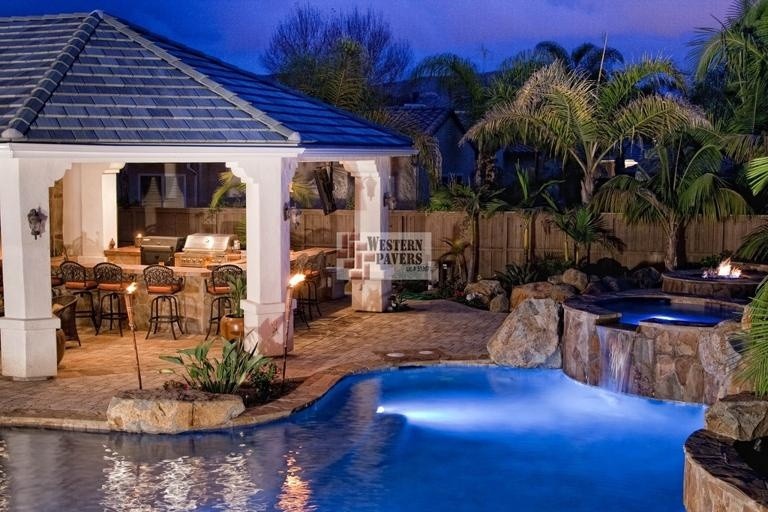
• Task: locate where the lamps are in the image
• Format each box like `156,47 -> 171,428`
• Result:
384,191 -> 396,212
27,209 -> 42,239
283,198 -> 302,229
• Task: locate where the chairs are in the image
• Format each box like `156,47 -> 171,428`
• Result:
51,262 -> 323,346
